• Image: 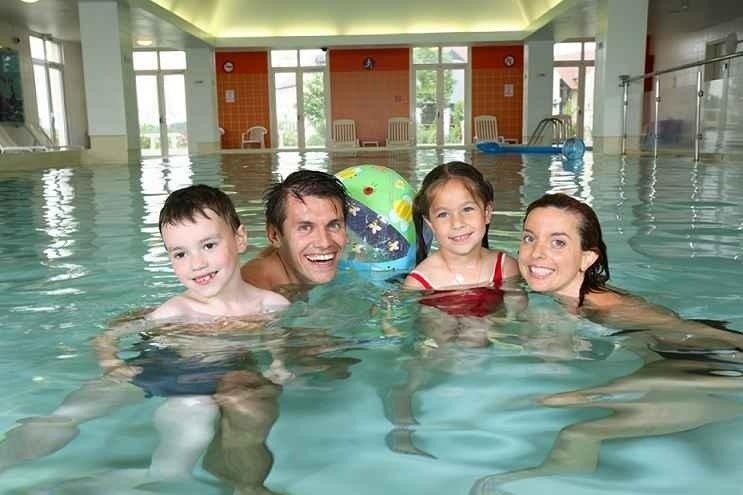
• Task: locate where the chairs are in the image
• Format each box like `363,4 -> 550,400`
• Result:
385,117 -> 414,146
0,123 -> 83,153
241,126 -> 268,148
332,119 -> 359,149
472,114 -> 504,144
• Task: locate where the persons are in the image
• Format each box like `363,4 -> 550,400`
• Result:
471,191 -> 743,495
400,159 -> 529,350
1,182 -> 293,484
110,170 -> 419,493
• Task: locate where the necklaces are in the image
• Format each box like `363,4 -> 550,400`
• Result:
439,249 -> 482,287
